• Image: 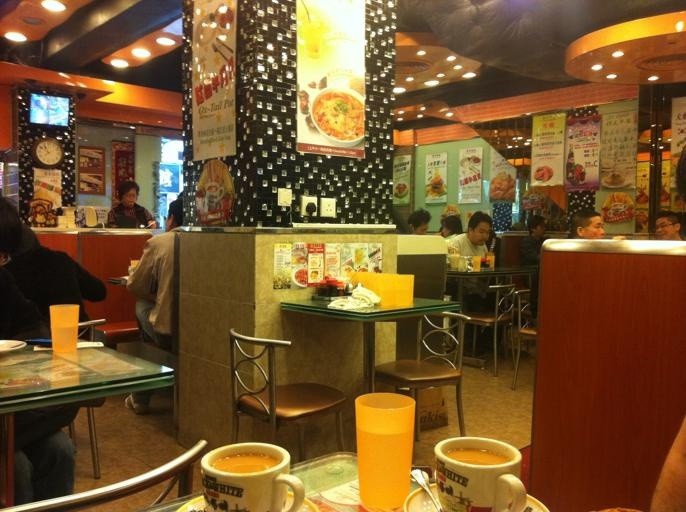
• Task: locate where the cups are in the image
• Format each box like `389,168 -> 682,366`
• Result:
57,215 -> 66,228
486,252 -> 495,267
130,259 -> 141,270
447,239 -> 462,271
434,435 -> 527,512
353,391 -> 417,512
49,304 -> 81,354
199,441 -> 305,512
51,352 -> 80,390
472,256 -> 481,272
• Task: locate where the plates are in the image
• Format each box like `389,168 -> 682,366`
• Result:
402,483 -> 549,512
174,491 -> 319,512
600,177 -> 633,189
0,339 -> 28,355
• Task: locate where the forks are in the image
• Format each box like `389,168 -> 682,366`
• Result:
211,43 -> 229,63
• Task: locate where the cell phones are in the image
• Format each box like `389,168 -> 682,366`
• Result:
26,335 -> 53,345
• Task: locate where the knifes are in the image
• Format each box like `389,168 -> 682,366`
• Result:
215,37 -> 234,54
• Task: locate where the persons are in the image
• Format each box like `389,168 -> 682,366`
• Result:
654,211 -> 684,240
0,190 -> 76,507
397,209 -> 433,235
530,211 -> 604,330
124,199 -> 183,415
107,181 -> 157,229
649,412 -> 686,512
441,215 -> 462,237
10,224 -> 117,348
521,215 -> 554,264
446,211 -> 505,357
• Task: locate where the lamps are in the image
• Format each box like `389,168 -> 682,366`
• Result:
395,32 -> 482,93
564,10 -> 686,84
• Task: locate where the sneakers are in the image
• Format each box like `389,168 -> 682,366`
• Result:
124,393 -> 151,412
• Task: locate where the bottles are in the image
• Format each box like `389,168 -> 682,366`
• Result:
464,256 -> 474,272
61,206 -> 76,229
317,276 -> 356,297
456,256 -> 468,272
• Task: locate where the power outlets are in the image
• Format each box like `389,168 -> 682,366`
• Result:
301,196 -> 317,216
320,198 -> 336,218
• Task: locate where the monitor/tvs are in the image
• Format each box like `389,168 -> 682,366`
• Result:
27,91 -> 70,129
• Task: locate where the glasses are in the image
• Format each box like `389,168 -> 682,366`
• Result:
655,223 -> 680,228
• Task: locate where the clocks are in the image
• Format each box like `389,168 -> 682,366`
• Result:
32,136 -> 64,168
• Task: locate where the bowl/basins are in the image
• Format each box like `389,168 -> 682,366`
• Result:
291,266 -> 307,287
309,86 -> 366,148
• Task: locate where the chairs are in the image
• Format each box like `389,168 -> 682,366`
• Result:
2,318 -> 106,488
455,283 -> 516,377
34,230 -> 78,261
0,439 -> 209,512
375,311 -> 472,441
229,327 -> 347,462
512,289 -> 537,391
76,231 -> 157,345
